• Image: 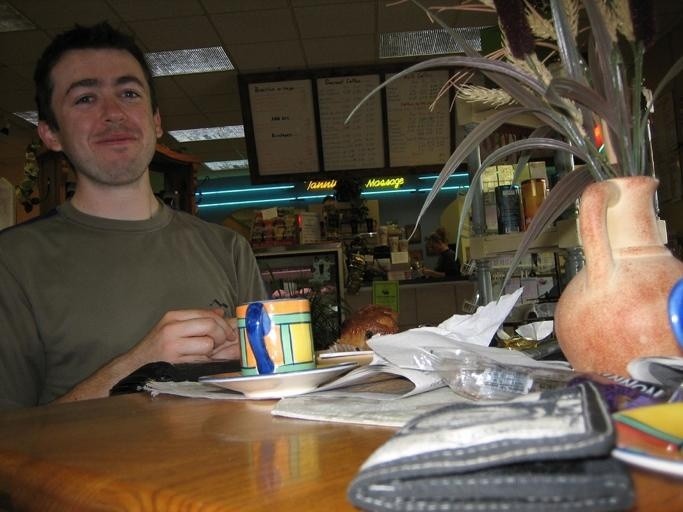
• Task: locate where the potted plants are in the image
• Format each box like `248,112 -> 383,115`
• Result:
342,0 -> 683,381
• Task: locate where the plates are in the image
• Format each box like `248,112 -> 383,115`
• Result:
199,362 -> 359,397
611,446 -> 683,486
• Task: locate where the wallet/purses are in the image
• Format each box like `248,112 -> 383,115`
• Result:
347,380 -> 635,511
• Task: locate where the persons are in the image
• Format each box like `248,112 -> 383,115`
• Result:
419,227 -> 461,278
0,21 -> 269,411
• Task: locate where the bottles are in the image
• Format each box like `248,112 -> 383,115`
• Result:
495,178 -> 547,235
564,247 -> 585,283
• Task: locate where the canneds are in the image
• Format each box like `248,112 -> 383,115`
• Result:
521,178 -> 546,231
495,184 -> 523,234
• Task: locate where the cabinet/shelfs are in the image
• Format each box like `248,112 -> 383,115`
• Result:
451,65 -> 668,362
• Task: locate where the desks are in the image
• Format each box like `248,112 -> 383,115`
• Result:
0,364 -> 683,512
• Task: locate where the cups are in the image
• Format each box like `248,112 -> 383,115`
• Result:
379,226 -> 409,252
236,300 -> 317,376
